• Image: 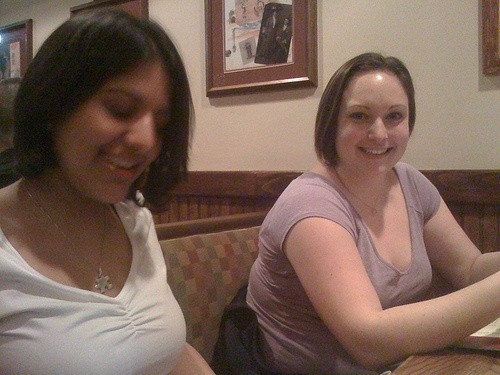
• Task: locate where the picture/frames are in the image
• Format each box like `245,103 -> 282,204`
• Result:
204,0 -> 318,97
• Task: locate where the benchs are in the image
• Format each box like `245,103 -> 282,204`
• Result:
155,212 -> 267,363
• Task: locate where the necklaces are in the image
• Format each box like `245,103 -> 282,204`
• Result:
335,164 -> 379,215
23,183 -> 113,293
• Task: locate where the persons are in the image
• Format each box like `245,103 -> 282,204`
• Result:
246,53 -> 500,375
0,8 -> 217,375
259,6 -> 292,60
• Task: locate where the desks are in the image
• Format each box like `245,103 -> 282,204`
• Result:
391,336 -> 500,375
